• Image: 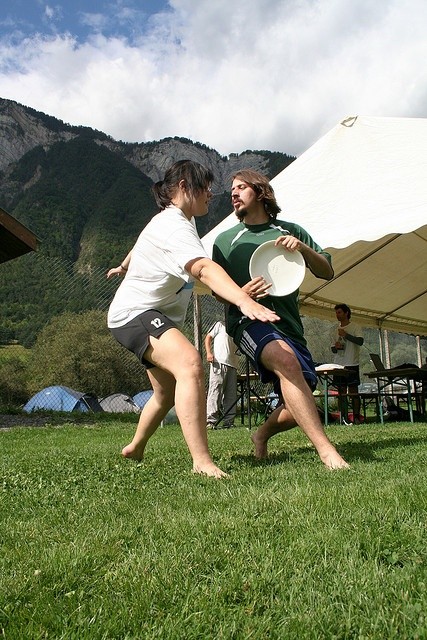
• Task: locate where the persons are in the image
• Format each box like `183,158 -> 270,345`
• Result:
211,169 -> 353,471
330,303 -> 364,425
204,315 -> 241,430
105,159 -> 282,481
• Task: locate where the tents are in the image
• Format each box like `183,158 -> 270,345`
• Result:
267,391 -> 283,411
22,385 -> 104,413
98,393 -> 141,413
131,390 -> 154,411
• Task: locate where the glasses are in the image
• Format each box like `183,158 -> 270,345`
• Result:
187,183 -> 212,193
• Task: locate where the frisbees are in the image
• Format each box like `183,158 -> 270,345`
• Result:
249,239 -> 307,297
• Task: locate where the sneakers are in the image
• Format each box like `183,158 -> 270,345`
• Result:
341,418 -> 353,426
223,423 -> 236,429
353,416 -> 360,426
205,422 -> 217,430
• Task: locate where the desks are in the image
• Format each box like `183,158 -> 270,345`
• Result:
364,368 -> 426,424
236,373 -> 267,424
314,370 -> 360,426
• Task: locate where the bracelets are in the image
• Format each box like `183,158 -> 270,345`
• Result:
119,264 -> 127,272
